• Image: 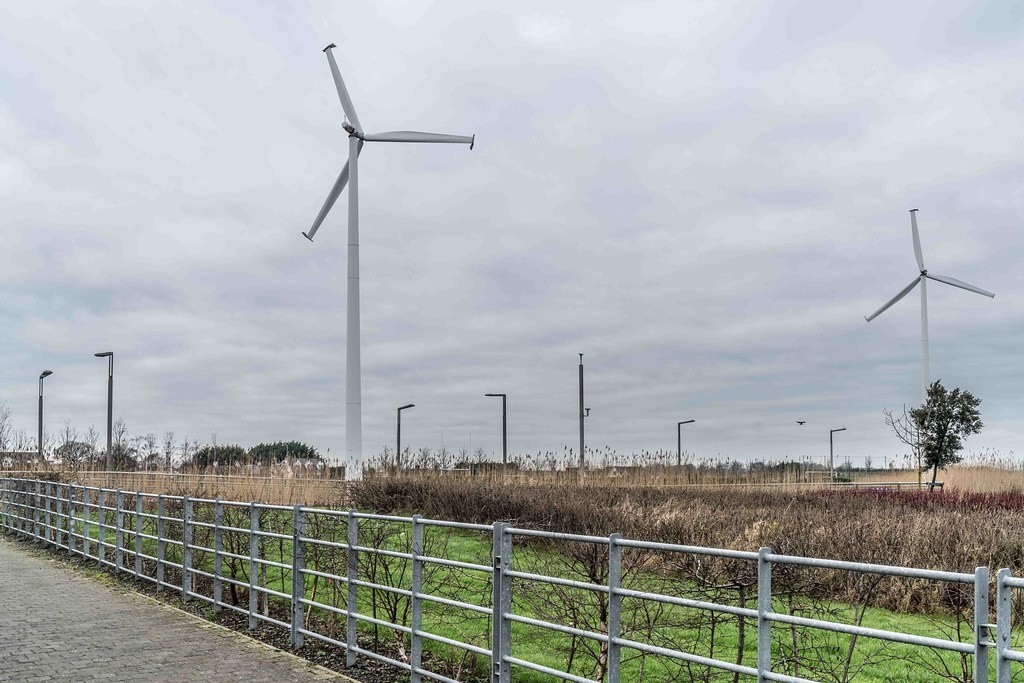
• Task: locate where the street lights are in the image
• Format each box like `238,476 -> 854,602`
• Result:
39,369 -> 52,475
486,394 -> 507,478
829,427 -> 846,483
94,351 -> 112,488
679,421 -> 695,478
397,404 -> 414,479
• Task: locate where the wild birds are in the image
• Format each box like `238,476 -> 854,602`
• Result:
796,419 -> 806,427
